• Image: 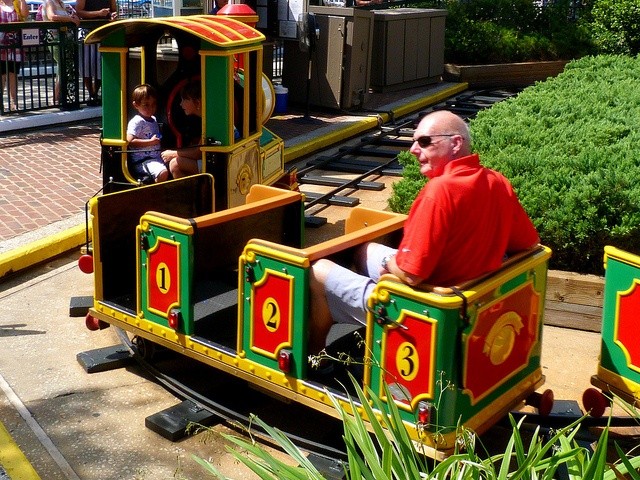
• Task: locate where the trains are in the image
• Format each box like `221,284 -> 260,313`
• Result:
81,5 -> 640,467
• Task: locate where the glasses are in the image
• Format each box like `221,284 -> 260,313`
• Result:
412,134 -> 465,148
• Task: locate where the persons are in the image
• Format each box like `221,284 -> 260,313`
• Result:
126,84 -> 169,183
42,0 -> 80,106
160,80 -> 241,179
309,110 -> 540,373
36,4 -> 44,44
75,0 -> 118,106
0,0 -> 25,113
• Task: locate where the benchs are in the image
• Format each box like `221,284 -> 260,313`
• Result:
238,206 -> 410,385
596,246 -> 640,408
362,244 -> 553,430
135,185 -> 305,337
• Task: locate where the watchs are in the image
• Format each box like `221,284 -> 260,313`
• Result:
381,257 -> 389,273
